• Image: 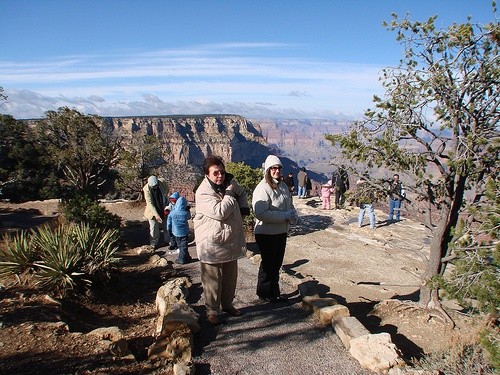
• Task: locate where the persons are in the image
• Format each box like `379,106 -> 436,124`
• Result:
355,174 -> 378,230
322,180 -> 334,210
332,165 -> 349,210
387,174 -> 406,225
252,155 -> 298,303
167,197 -> 192,265
297,167 -> 308,199
194,156 -> 250,326
283,172 -> 295,192
164,192 -> 180,250
143,176 -> 171,249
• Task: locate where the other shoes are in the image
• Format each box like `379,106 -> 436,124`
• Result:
208,315 -> 220,325
260,296 -> 281,303
224,307 -> 242,315
276,295 -> 287,301
168,245 -> 174,250
175,259 -> 180,263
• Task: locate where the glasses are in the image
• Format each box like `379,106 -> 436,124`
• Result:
210,170 -> 226,176
270,167 -> 282,170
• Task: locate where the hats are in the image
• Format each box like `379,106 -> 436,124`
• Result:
169,198 -> 176,202
148,176 -> 158,186
